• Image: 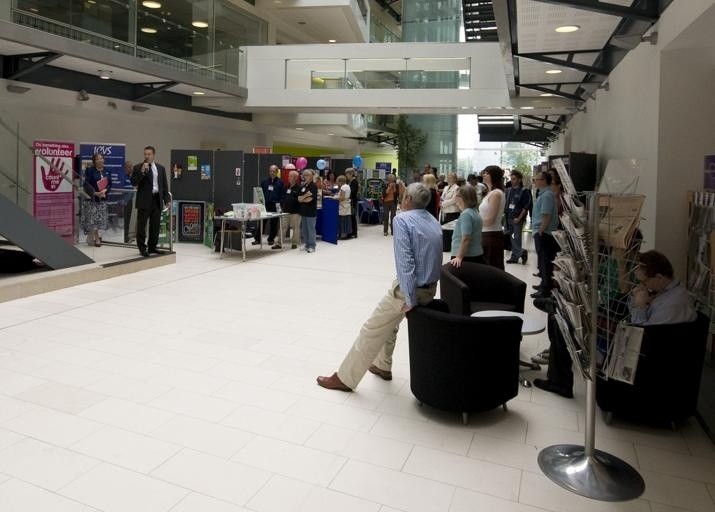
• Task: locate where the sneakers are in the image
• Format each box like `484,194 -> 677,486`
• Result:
252,239 -> 315,252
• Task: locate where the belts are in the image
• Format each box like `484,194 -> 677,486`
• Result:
418,283 -> 437,289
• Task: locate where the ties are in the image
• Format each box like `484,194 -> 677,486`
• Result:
149,163 -> 153,189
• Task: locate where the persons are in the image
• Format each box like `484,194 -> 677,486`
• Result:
532,168 -> 568,277
380,174 -> 399,236
529,172 -> 558,297
316,183 -> 444,392
130,146 -> 170,256
251,165 -> 358,253
84,153 -> 112,247
118,161 -> 134,243
412,164 -> 532,271
532,249 -> 696,398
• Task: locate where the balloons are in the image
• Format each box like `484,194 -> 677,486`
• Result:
352,155 -> 361,168
295,156 -> 307,170
316,159 -> 327,171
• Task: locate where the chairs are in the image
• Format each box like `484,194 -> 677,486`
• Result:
360,198 -> 380,224
405,299 -> 520,423
596,314 -> 712,432
440,261 -> 528,316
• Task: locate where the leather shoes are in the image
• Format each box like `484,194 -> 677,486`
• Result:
317,373 -> 352,391
148,248 -> 164,254
369,364 -> 392,380
507,250 -> 573,398
141,250 -> 150,257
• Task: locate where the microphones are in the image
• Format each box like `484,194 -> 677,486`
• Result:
143,158 -> 151,177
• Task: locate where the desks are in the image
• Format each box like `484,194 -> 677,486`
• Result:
214,211 -> 290,263
